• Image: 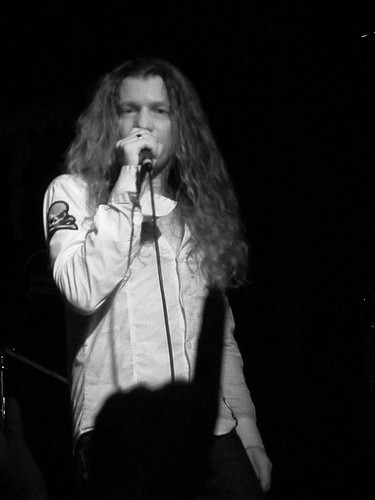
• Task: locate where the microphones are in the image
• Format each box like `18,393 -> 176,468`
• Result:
139,148 -> 154,172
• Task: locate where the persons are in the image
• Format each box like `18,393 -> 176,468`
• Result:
44,55 -> 271,498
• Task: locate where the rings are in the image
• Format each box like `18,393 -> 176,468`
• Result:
137,134 -> 142,140
